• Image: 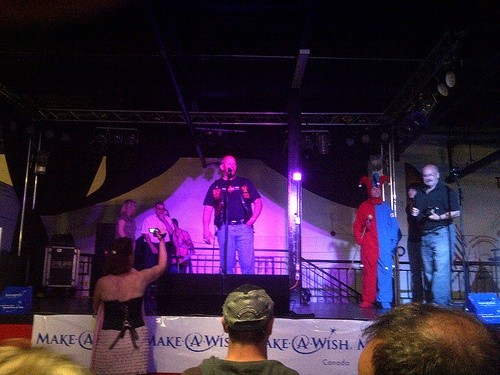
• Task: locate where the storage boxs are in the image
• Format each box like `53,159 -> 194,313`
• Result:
43,245 -> 81,291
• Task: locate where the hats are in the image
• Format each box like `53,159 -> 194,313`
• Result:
359,173 -> 391,198
222,284 -> 274,331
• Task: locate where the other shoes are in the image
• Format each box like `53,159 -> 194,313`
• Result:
359,302 -> 374,307
382,302 -> 391,308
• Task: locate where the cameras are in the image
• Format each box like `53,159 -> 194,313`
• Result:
149,227 -> 159,233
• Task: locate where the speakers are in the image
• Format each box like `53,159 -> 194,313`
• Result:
156,272 -> 290,315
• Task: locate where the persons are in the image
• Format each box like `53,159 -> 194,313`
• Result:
358,303 -> 500,375
411,164 -> 460,308
90,228 -> 167,375
183,284 -> 300,375
353,173 -> 402,309
141,201 -> 175,271
115,199 -> 138,251
405,182 -> 432,306
170,218 -> 196,274
203,155 -> 264,274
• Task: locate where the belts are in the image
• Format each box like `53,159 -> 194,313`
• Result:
223,220 -> 247,225
419,225 -> 445,235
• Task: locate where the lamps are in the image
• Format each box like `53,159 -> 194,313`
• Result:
431,64 -> 464,97
